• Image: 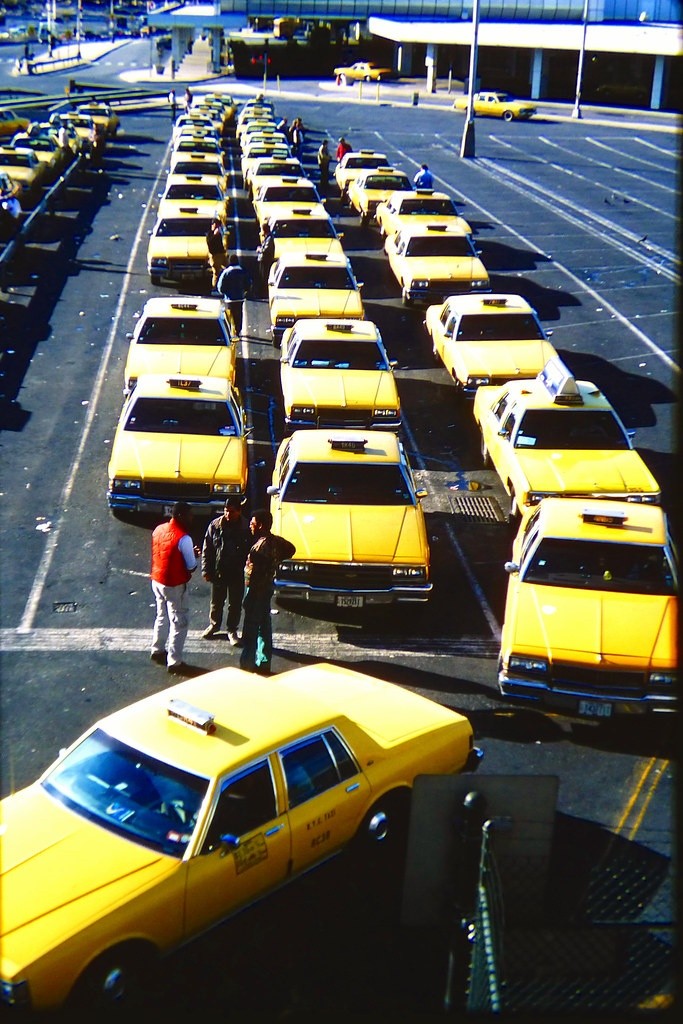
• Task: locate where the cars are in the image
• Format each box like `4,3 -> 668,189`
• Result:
373,188 -> 466,241
265,426 -> 434,610
334,61 -> 394,83
258,206 -> 346,262
266,252 -> 366,348
0,660 -> 485,1024
104,372 -> 255,518
154,92 -> 328,225
454,91 -> 539,122
496,499 -> 681,721
146,205 -> 231,286
472,355 -> 662,520
423,293 -> 560,398
346,166 -> 416,227
0,99 -> 121,229
121,297 -> 241,398
383,224 -> 493,307
332,149 -> 391,202
279,319 -> 405,432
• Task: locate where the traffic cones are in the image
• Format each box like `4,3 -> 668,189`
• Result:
334,75 -> 341,86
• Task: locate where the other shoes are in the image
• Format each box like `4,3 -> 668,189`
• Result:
203,623 -> 219,638
168,661 -> 199,676
228,631 -> 241,648
150,650 -> 169,661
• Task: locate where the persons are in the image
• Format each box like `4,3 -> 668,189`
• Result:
50,118 -> 75,168
0,180 -> 24,201
202,496 -> 257,646
335,137 -> 353,163
289,116 -> 305,162
149,501 -> 202,673
169,88 -> 178,122
257,92 -> 263,104
414,163 -> 434,188
184,87 -> 192,114
277,115 -> 287,131
88,122 -> 105,174
317,139 -> 332,191
241,508 -> 295,675
